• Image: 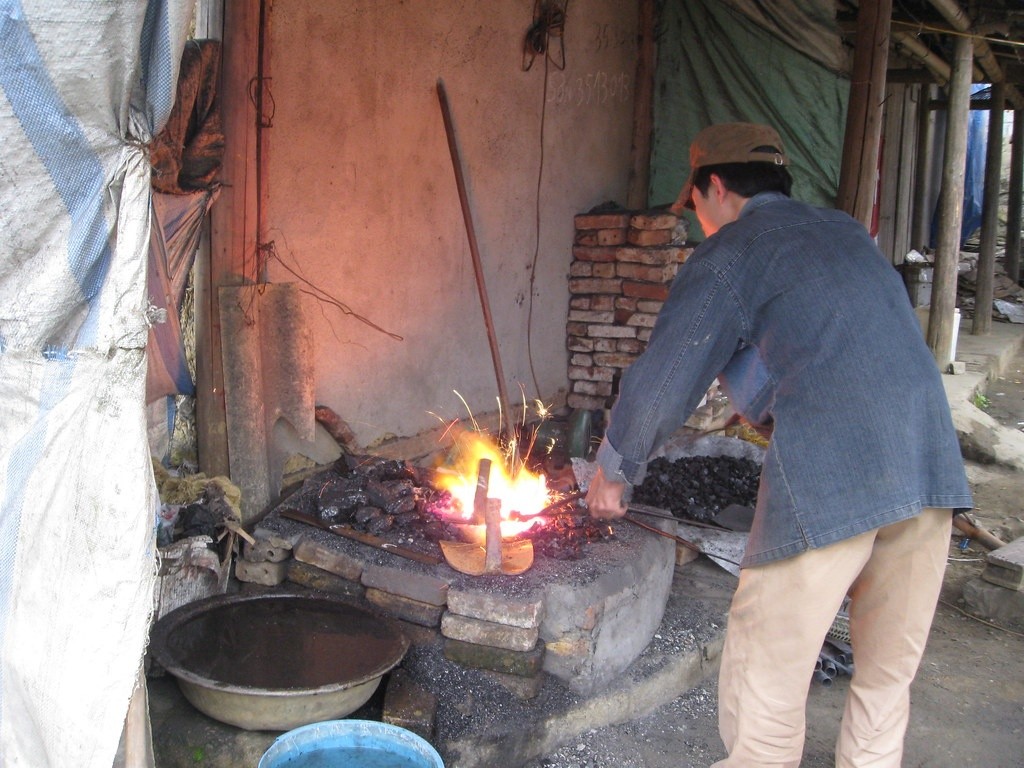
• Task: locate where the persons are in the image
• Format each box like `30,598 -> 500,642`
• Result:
577,122 -> 976,768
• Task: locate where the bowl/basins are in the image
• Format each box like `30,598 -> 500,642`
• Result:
148,590 -> 412,732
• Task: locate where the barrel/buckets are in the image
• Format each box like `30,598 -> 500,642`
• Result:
257,719 -> 445,768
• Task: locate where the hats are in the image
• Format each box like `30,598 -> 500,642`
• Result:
673,122 -> 789,212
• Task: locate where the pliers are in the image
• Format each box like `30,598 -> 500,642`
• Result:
508,490 -> 587,522
